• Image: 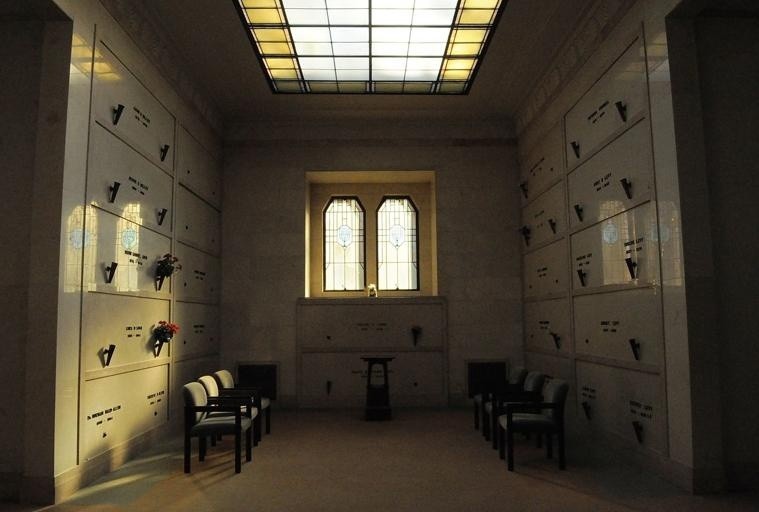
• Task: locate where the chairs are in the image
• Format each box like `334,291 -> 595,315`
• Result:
182,370 -> 271,474
472,366 -> 569,471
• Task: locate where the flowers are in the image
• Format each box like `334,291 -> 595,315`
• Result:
155,320 -> 180,356
156,253 -> 182,289
411,325 -> 424,346
